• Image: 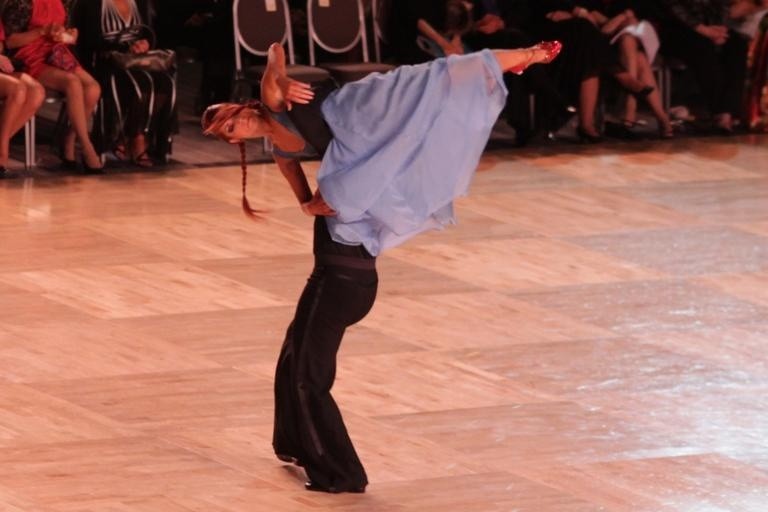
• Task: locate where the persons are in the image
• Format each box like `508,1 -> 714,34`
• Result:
194,1 -> 234,117
199,38 -> 565,226
0,16 -> 48,170
1,0 -> 105,174
69,1 -> 177,169
387,0 -> 768,149
267,0 -> 474,494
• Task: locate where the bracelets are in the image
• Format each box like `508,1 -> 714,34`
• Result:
40,26 -> 47,37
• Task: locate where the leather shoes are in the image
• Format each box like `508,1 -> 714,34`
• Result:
510,40 -> 563,76
514,118 -> 674,147
56,132 -> 103,172
277,453 -> 364,492
632,82 -> 654,99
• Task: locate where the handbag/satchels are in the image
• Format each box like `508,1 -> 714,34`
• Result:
46,43 -> 76,70
110,49 -> 174,70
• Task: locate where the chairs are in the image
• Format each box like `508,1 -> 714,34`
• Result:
227,1 -> 398,157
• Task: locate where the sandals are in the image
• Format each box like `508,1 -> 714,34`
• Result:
113,132 -> 154,167
